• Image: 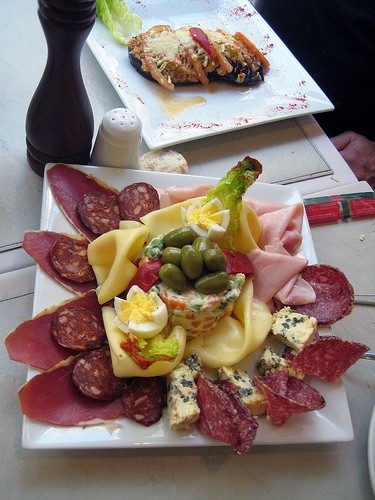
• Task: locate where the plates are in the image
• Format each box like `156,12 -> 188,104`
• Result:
86,0 -> 335,151
22,162 -> 355,450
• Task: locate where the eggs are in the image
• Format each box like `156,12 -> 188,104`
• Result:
178,197 -> 231,244
113,284 -> 168,340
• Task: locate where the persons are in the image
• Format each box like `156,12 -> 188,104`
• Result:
256,0 -> 375,184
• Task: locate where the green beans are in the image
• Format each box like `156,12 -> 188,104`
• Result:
159,228 -> 228,293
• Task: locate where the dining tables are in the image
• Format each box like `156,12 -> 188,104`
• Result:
2,0 -> 357,274
0,179 -> 374,500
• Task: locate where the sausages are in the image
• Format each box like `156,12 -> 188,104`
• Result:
76,181 -> 160,235
50,304 -> 165,427
48,235 -> 94,283
